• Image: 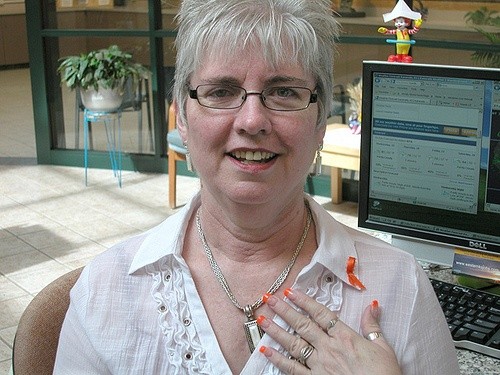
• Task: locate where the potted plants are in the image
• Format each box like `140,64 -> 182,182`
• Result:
58,45 -> 137,115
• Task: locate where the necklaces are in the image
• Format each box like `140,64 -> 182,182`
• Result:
196,199 -> 312,353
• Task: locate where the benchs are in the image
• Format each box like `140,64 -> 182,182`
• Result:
312,124 -> 362,203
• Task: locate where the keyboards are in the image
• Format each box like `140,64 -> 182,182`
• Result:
427,276 -> 500,360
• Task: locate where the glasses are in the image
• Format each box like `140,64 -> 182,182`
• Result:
188,83 -> 317,111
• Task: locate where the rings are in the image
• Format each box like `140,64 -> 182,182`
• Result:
367,332 -> 383,340
325,317 -> 339,333
298,345 -> 314,365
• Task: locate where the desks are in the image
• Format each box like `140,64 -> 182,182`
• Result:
83,102 -> 141,189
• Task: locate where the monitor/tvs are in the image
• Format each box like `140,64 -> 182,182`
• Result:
357,60 -> 500,295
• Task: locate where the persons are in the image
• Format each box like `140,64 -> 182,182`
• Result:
378,17 -> 423,63
51,0 -> 462,375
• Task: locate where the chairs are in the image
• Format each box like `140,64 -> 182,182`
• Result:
11,267 -> 87,375
166,99 -> 197,209
